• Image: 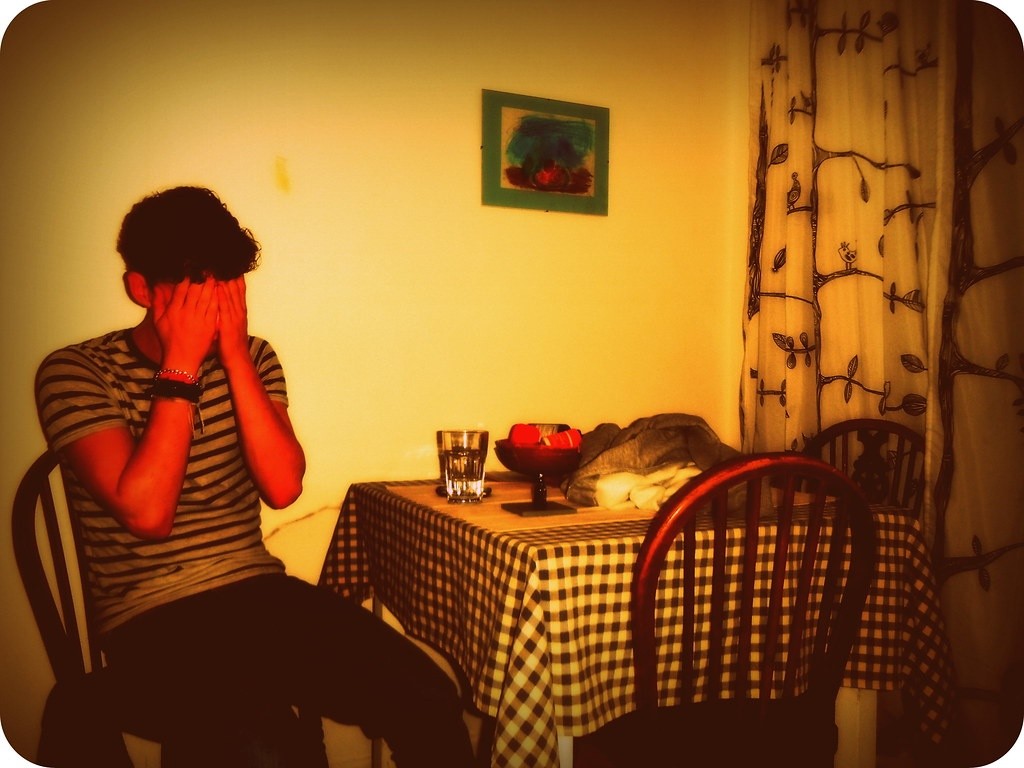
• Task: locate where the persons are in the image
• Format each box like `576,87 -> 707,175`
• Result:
35,186 -> 475,768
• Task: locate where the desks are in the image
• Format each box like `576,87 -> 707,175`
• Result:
317,473 -> 963,768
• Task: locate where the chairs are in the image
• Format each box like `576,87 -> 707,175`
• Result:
10,452 -> 327,768
799,420 -> 929,509
610,452 -> 878,768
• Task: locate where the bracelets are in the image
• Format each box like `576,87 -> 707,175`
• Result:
152,368 -> 205,439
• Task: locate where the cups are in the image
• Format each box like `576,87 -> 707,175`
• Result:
438,430 -> 489,503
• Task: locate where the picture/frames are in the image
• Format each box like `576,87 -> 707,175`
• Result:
481,89 -> 611,216
